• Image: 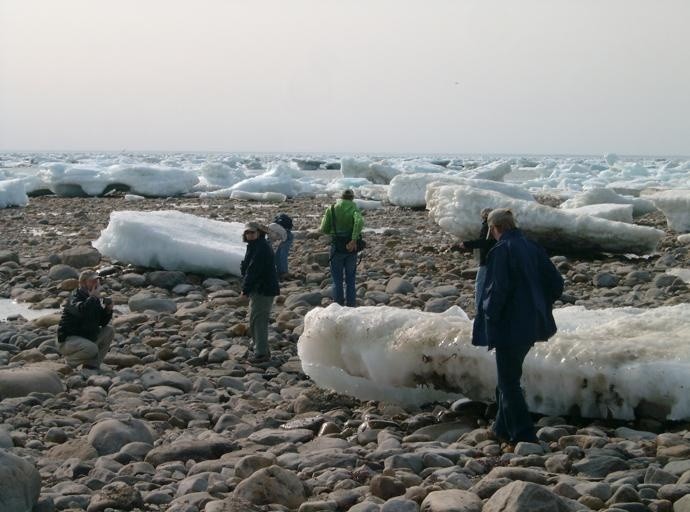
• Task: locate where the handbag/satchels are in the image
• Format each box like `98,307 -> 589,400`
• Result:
334,236 -> 366,254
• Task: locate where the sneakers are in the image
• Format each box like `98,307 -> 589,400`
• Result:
476,430 -> 509,441
248,350 -> 272,362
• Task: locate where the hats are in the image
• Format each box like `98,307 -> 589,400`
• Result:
486,209 -> 513,239
274,213 -> 293,226
79,270 -> 101,281
242,220 -> 270,241
342,189 -> 354,201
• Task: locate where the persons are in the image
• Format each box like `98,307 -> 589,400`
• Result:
460,207 -> 495,317
318,190 -> 365,307
240,222 -> 280,363
56,270 -> 114,374
473,208 -> 565,444
261,213 -> 294,275
